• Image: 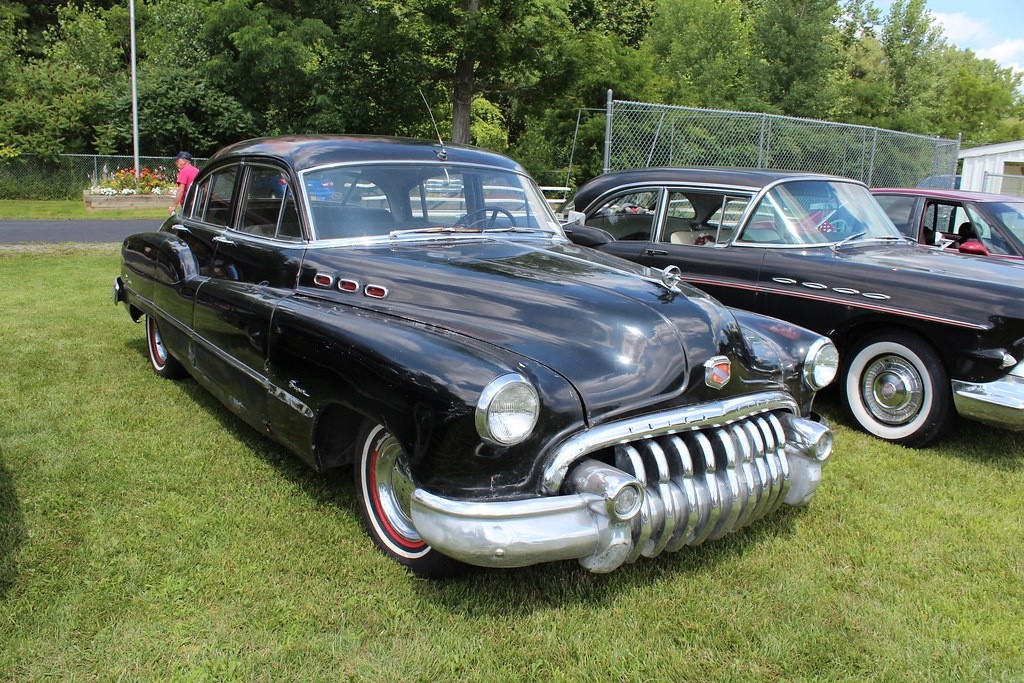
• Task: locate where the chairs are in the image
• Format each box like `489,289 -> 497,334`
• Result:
919,226 -> 943,246
957,221 -> 1001,255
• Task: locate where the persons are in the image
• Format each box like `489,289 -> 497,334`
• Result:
169,152 -> 200,217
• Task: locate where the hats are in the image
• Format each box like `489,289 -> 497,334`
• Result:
173,151 -> 192,160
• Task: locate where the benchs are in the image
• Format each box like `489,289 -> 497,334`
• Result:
205,204 -> 437,245
586,214 -> 698,241
665,228 -> 781,245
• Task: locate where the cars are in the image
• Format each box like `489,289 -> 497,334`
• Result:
113,132 -> 841,579
270,173 -> 335,203
916,175 -> 961,190
732,187 -> 1024,264
548,166 -> 1024,450
